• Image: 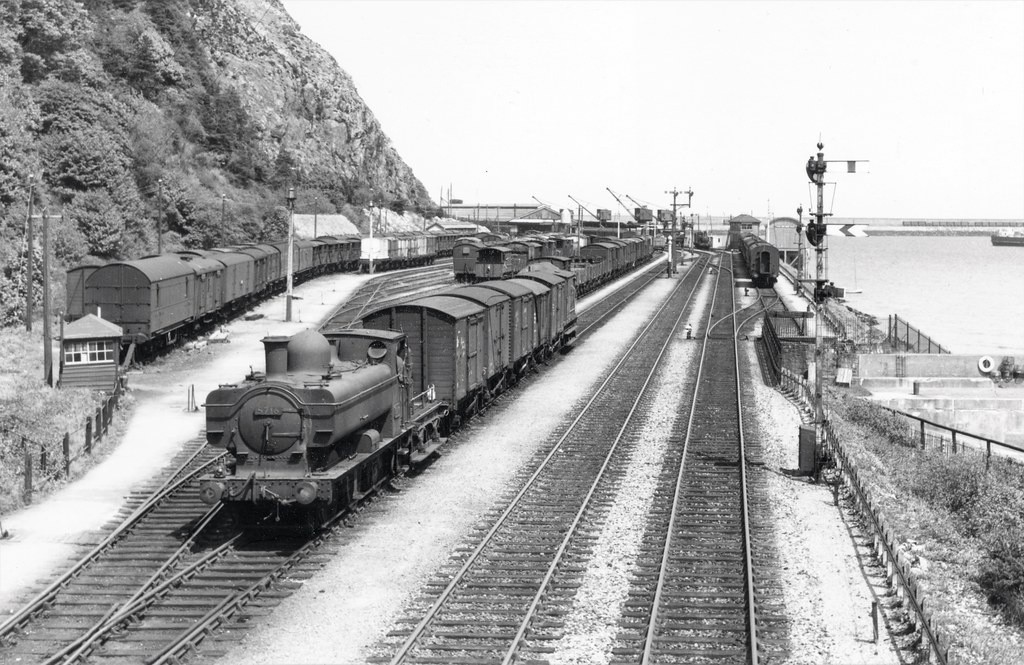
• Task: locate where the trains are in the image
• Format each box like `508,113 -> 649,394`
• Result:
61,226 -> 591,365
196,235 -> 653,534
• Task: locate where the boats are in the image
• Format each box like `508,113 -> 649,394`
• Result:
991,229 -> 1024,247
